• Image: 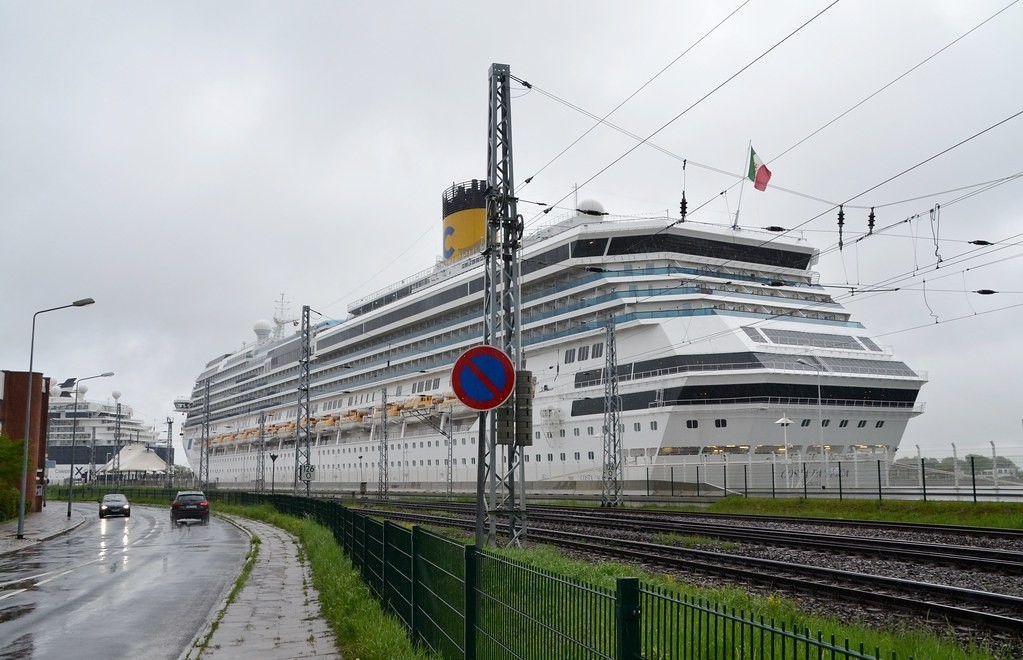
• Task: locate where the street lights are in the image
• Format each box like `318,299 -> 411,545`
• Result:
67,371 -> 115,518
16,296 -> 95,541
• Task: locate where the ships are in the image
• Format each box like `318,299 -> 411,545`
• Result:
43,378 -> 176,484
179,168 -> 930,499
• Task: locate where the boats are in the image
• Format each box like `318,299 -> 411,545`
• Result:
277,419 -> 298,437
374,401 -> 404,417
343,407 -> 371,423
212,435 -> 223,444
239,429 -> 251,440
317,414 -> 341,430
403,394 -> 439,412
250,426 -> 262,438
221,434 -> 235,443
261,423 -> 281,437
297,417 -> 317,432
433,394 -> 463,411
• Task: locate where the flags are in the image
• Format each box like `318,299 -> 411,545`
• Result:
748,146 -> 772,191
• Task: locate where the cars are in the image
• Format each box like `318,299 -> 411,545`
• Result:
98,493 -> 133,519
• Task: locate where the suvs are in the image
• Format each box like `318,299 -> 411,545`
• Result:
170,490 -> 210,524
